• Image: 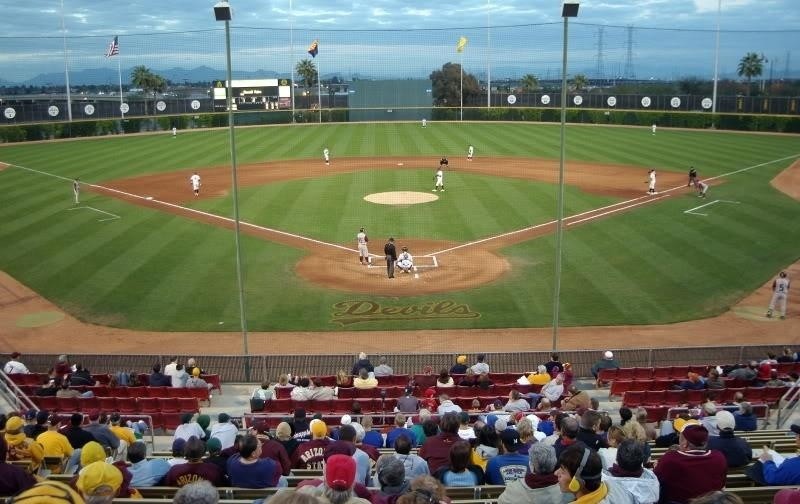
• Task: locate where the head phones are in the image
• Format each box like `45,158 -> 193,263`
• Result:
569,448 -> 591,493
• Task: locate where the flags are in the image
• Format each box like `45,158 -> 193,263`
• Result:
456,37 -> 467,53
309,41 -> 319,56
105,36 -> 120,58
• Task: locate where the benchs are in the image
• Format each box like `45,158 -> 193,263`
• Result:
1,449 -> 557,502
1,373 -> 223,432
595,362 -> 800,423
647,428 -> 798,502
244,372 -> 609,435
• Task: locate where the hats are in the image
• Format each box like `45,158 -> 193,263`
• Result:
791,424 -> 800,434
404,386 -> 414,395
325,454 -> 356,489
704,402 -> 715,414
376,455 -> 406,487
674,418 -> 709,446
11,352 -> 20,358
538,365 -> 547,374
179,406 -> 353,443
457,355 -> 468,364
460,402 -> 598,438
716,410 -> 735,431
687,372 -> 700,384
424,389 -> 436,397
605,350 -> 614,359
193,367 -> 200,376
6,407 -> 121,504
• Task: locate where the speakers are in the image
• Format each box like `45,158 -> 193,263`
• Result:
213,2 -> 232,21
560,1 -> 579,18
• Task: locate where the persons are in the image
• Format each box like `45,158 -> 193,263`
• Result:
172,125 -> 176,138
432,156 -> 449,192
687,166 -> 708,200
190,172 -> 202,197
384,237 -> 397,278
652,122 -> 657,134
74,177 -> 81,204
323,146 -> 330,166
396,246 -> 414,274
646,169 -> 656,193
357,228 -> 372,264
421,117 -> 427,128
468,144 -> 473,160
766,272 -> 790,319
